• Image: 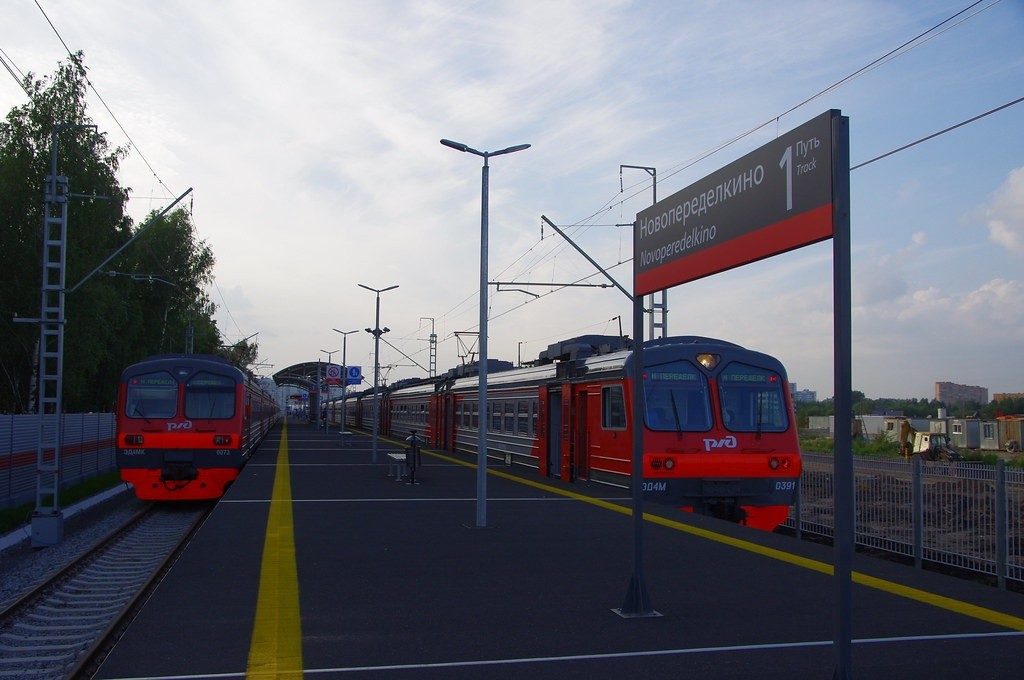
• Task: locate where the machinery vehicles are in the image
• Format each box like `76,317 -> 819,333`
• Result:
899,419 -> 963,474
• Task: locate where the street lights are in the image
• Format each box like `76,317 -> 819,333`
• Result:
332,328 -> 359,433
439,139 -> 531,528
320,349 -> 341,434
357,283 -> 400,464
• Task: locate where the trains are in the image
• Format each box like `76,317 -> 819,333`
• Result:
321,335 -> 803,533
114,354 -> 281,501
